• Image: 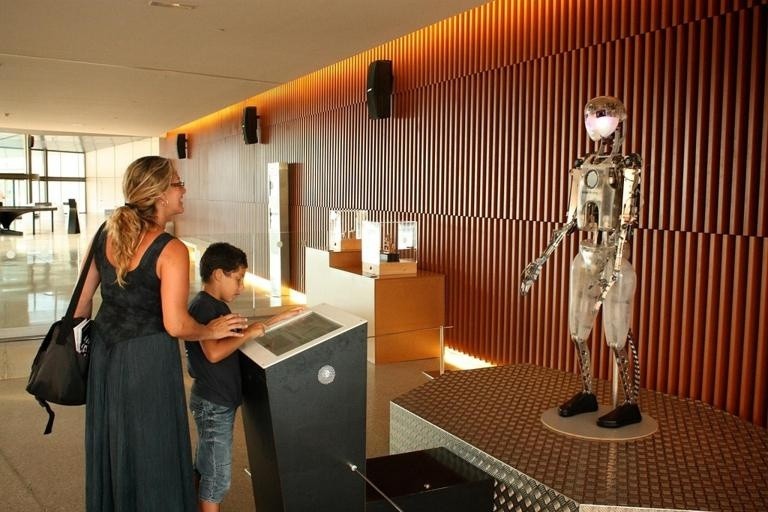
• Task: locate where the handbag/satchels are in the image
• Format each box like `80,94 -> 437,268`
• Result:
26,317 -> 95,405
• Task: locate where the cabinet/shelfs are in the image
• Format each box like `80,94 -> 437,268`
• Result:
305,244 -> 444,365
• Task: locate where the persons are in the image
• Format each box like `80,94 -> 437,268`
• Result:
520,97 -> 643,429
184,243 -> 306,512
73,156 -> 248,511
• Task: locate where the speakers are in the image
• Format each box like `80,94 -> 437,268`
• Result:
366,59 -> 393,120
241,106 -> 259,145
176,133 -> 187,159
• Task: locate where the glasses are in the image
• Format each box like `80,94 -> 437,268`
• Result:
171,182 -> 184,187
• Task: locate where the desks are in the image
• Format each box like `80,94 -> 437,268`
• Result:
0,206 -> 56,234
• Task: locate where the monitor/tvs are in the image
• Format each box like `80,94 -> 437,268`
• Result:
254,309 -> 343,357
